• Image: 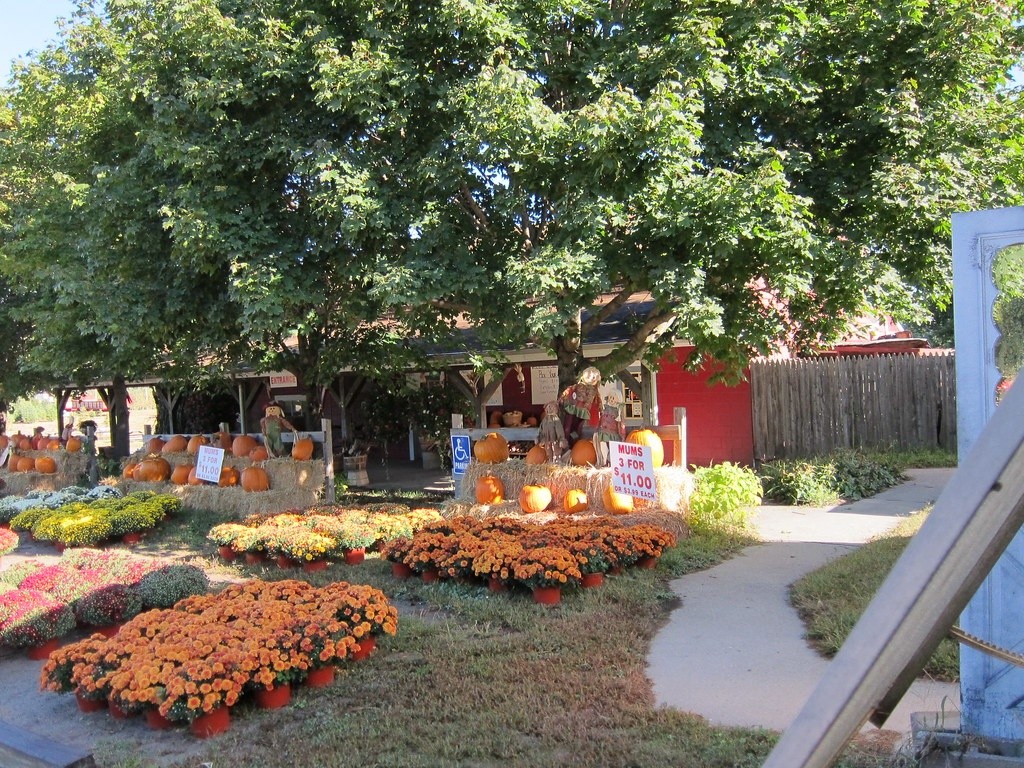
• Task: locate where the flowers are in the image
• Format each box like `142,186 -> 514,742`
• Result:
0,486 -> 183,558
206,503 -> 445,561
0,548 -> 210,647
39,579 -> 398,722
381,484 -> 675,587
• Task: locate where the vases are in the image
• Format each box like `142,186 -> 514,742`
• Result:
219,546 -> 235,562
303,559 -> 327,573
146,708 -> 172,728
120,532 -> 140,546
344,547 -> 365,566
93,621 -> 124,639
55,540 -> 67,552
79,542 -> 98,549
259,681 -> 291,710
26,530 -> 36,543
490,578 -> 503,592
609,568 -> 622,577
456,575 -> 473,586
245,552 -> 265,566
350,636 -> 375,662
531,587 -> 560,605
304,662 -> 334,689
377,539 -> 386,553
640,556 -> 655,570
76,694 -> 104,712
392,563 -> 409,581
107,697 -> 135,720
189,701 -> 230,739
26,637 -> 58,660
423,569 -> 439,583
276,554 -> 296,570
585,572 -> 604,588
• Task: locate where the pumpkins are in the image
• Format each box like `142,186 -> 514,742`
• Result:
572,438 -> 597,467
602,485 -> 633,515
475,470 -> 505,505
474,433 -> 509,464
518,479 -> 552,513
625,423 -> 664,468
487,409 -> 545,428
122,429 -> 313,492
524,445 -> 549,465
0,430 -> 81,473
563,488 -> 589,514
